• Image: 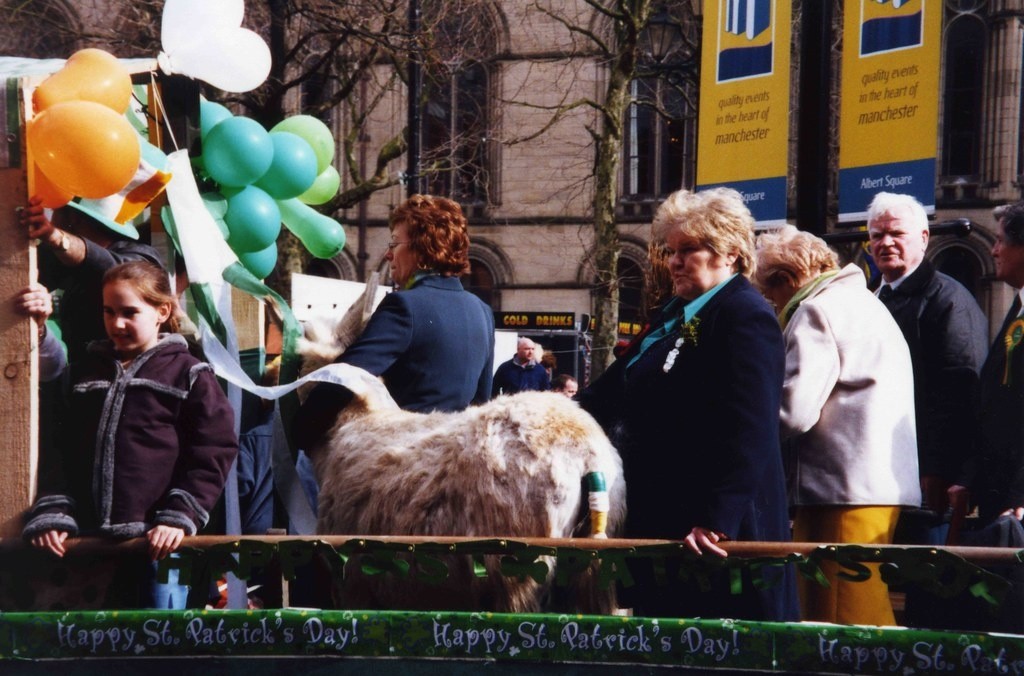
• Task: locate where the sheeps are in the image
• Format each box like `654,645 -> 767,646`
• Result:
289,363 -> 628,614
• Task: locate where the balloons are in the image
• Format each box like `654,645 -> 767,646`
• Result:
27,0 -> 347,279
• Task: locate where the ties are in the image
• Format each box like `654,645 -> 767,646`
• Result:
878,285 -> 892,300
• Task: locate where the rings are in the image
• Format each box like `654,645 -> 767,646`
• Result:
41,298 -> 47,307
42,307 -> 47,316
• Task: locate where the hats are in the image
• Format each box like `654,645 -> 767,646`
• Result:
67,198 -> 140,240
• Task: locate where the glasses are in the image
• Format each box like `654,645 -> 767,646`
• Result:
388,242 -> 412,249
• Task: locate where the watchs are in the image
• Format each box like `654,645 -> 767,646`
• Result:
52,228 -> 70,256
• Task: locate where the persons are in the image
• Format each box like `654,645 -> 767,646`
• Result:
866,189 -> 990,629
947,200 -> 1024,636
569,180 -> 805,624
14,191 -> 176,429
291,191 -> 495,468
235,350 -> 330,542
20,259 -> 239,611
490,336 -> 581,403
752,221 -> 924,627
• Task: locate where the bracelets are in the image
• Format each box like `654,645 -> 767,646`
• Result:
39,326 -> 45,341
